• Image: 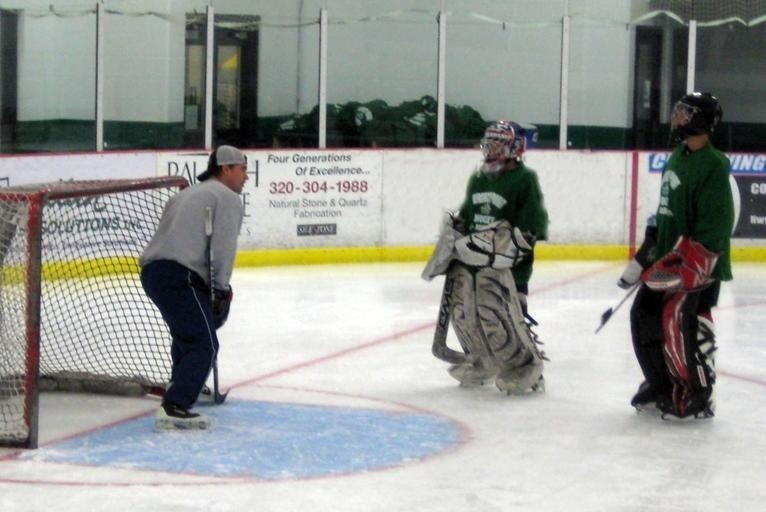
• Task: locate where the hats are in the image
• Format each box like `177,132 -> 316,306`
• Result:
196,144 -> 248,181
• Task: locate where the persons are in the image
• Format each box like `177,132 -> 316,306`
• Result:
617,88 -> 733,417
136,143 -> 251,426
419,118 -> 549,394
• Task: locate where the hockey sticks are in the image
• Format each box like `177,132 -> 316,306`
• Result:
204,207 -> 230,405
594,251 -> 656,333
430,218 -> 480,367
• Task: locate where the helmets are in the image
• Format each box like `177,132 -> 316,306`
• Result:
484,119 -> 528,159
679,90 -> 724,138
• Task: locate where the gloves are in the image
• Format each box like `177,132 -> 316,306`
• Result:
211,284 -> 233,330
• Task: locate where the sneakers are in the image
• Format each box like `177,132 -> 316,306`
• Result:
164,376 -> 213,398
659,396 -> 716,422
630,380 -> 673,407
154,399 -> 213,424
